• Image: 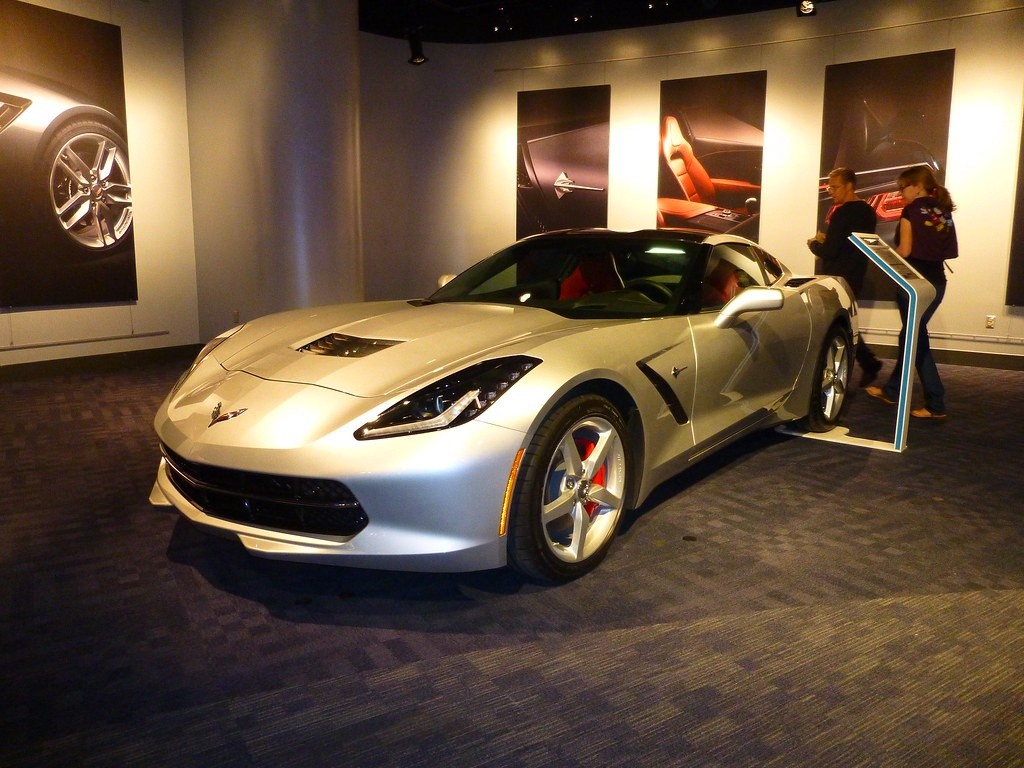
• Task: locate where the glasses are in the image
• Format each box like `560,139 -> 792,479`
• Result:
826,183 -> 846,193
899,183 -> 912,193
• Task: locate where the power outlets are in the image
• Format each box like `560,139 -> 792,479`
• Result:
232,310 -> 239,323
985,315 -> 995,329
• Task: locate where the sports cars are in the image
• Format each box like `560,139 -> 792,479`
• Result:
148,227 -> 860,588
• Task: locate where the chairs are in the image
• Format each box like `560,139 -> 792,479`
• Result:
662,116 -> 759,215
555,257 -> 616,302
702,263 -> 739,308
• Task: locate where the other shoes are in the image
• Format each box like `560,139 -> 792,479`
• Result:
860,358 -> 882,387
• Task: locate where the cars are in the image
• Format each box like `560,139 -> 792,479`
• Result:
0,58 -> 131,260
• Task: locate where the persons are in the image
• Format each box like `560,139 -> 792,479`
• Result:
807,168 -> 877,388
865,165 -> 958,420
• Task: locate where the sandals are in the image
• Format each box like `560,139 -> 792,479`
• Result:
865,386 -> 897,404
912,407 -> 947,418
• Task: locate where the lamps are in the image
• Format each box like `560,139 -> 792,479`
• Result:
796,0 -> 817,18
401,23 -> 430,67
